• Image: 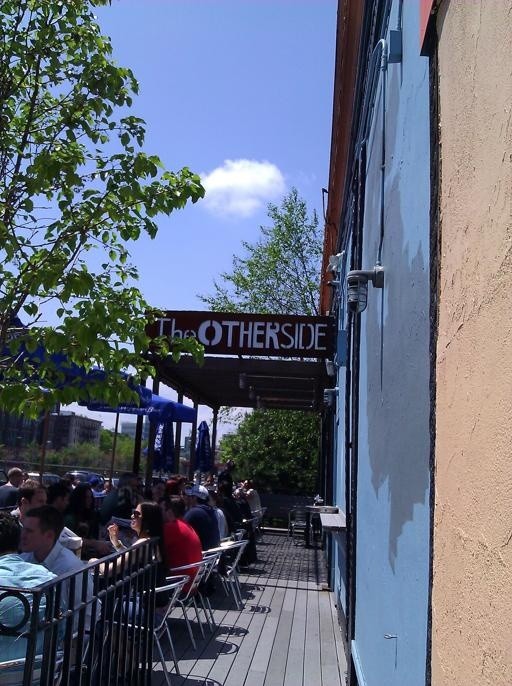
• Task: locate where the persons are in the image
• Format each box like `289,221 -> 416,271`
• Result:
1,460 -> 261,686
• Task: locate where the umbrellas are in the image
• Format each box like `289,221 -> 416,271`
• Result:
0,323 -> 214,482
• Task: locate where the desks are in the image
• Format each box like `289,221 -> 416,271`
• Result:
306,506 -> 338,550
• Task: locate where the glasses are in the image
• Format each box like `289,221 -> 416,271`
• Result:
132,508 -> 142,519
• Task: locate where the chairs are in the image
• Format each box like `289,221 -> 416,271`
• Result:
288,504 -> 314,545
0,507 -> 269,686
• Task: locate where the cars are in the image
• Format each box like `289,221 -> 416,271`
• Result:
102,478 -> 120,488
64,470 -> 95,484
27,472 -> 66,487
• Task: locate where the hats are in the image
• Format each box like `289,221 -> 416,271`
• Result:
185,485 -> 209,500
